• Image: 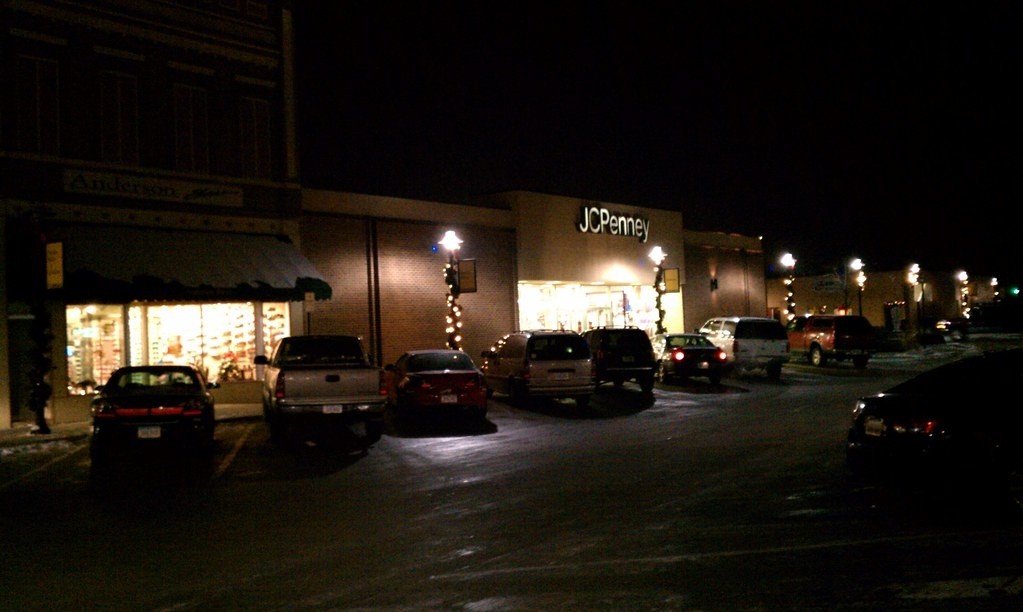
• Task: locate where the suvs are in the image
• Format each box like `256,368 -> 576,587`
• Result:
583,325 -> 657,394
480,331 -> 596,410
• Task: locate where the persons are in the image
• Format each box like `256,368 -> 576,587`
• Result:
28,369 -> 50,433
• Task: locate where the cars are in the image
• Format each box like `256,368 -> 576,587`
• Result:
650,333 -> 726,384
692,316 -> 791,381
846,348 -> 1023,505
90,365 -> 220,459
384,349 -> 489,424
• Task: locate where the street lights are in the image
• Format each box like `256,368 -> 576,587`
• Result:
437,230 -> 466,351
906,264 -> 923,333
956,270 -> 970,319
779,252 -> 797,320
649,246 -> 668,334
849,258 -> 867,316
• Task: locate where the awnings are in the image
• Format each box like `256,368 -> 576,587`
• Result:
0,219 -> 332,304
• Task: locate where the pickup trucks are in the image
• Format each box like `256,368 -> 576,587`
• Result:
785,315 -> 875,368
254,335 -> 389,442
